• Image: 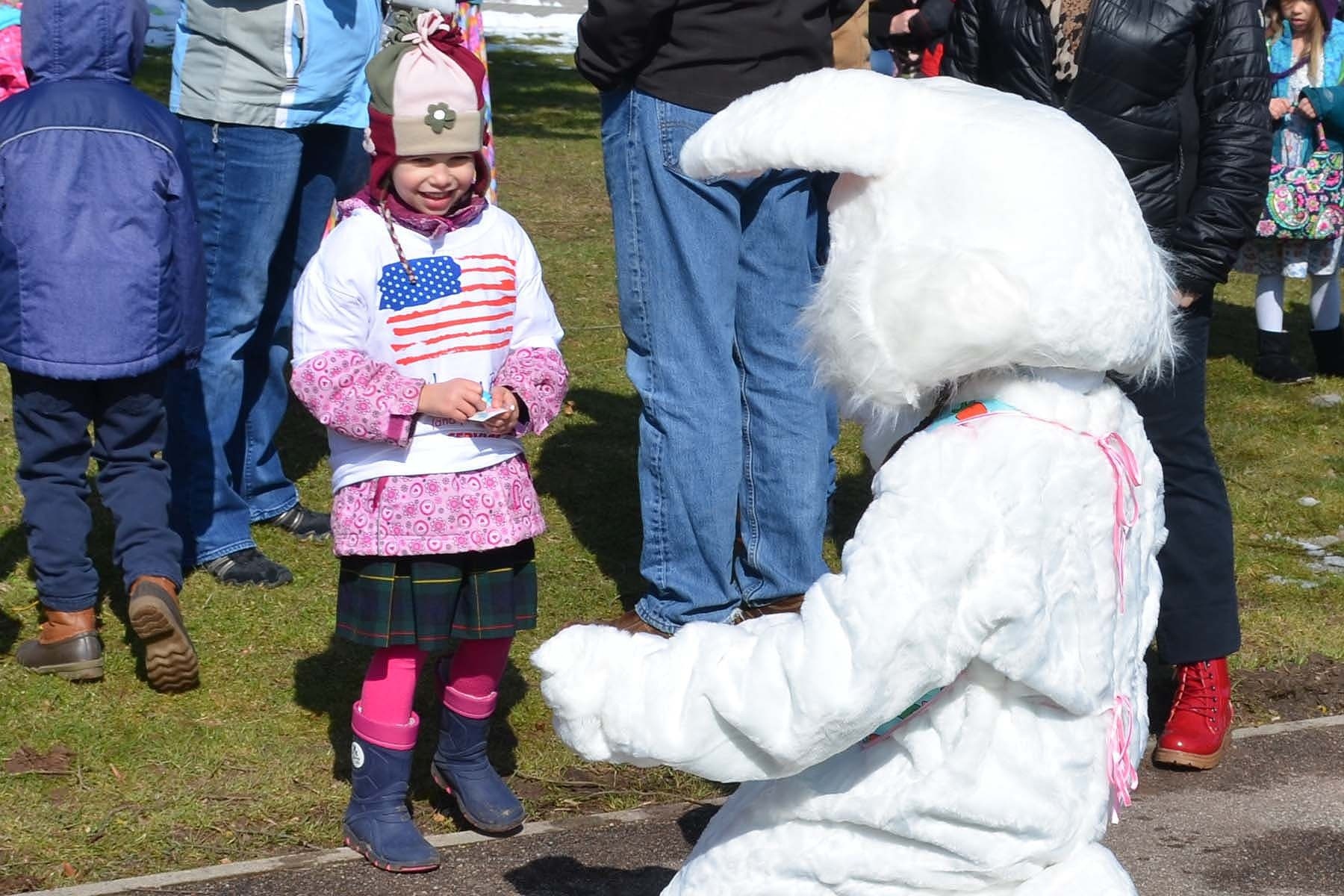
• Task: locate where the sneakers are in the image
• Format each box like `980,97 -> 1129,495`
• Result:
199,545 -> 293,591
252,500 -> 333,540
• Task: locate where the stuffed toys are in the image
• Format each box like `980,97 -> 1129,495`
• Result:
530,66 -> 1190,896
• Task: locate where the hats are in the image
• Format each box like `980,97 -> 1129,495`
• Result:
363,7 -> 492,201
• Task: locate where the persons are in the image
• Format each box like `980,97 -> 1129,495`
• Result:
168,0 -> 382,588
943,0 -> 1272,770
867,0 -> 955,75
573,0 -> 868,638
0,0 -> 208,696
290,8 -> 569,872
1232,0 -> 1344,386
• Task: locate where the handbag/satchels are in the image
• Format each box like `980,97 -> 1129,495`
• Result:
1249,117 -> 1344,243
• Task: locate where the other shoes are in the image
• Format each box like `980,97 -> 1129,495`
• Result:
559,606 -> 674,641
731,592 -> 807,625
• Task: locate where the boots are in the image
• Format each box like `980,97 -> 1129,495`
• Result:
1152,657 -> 1236,770
1251,327 -> 1310,384
15,607 -> 104,684
340,700 -> 441,873
419,682 -> 526,834
127,574 -> 201,696
1306,323 -> 1344,378
